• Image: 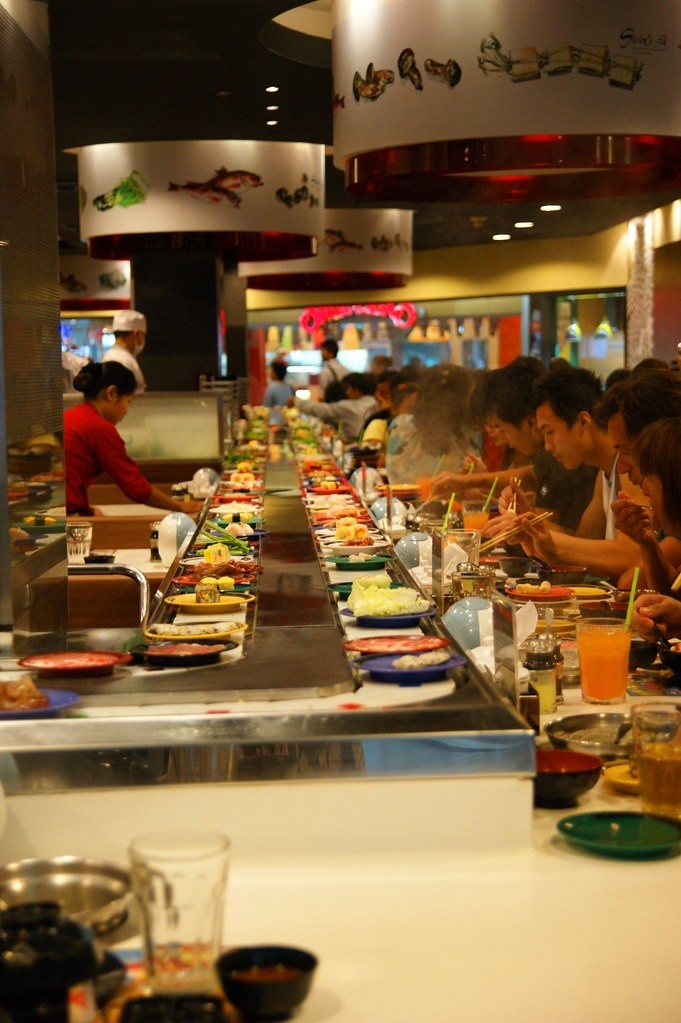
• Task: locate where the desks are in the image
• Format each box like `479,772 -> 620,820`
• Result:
67,502 -> 172,549
64,548 -> 170,574
26,557 -> 69,577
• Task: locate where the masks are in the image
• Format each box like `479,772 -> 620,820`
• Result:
135,334 -> 145,354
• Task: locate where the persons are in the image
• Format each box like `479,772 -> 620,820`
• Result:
285,354 -> 681,645
98,311 -> 148,396
319,340 -> 349,397
271,348 -> 289,367
64,361 -> 204,516
264,362 -> 293,408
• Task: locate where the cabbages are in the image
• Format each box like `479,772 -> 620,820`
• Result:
347,581 -> 430,617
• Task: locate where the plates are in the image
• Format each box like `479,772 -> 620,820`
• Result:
558,811 -> 681,856
0,412 -> 681,757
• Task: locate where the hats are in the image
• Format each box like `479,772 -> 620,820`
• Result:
113,310 -> 147,332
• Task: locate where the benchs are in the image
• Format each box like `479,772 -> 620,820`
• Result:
29,578 -> 67,631
67,575 -> 167,630
89,483 -> 173,504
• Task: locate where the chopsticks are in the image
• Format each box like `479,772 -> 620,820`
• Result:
507,477 -> 521,517
476,511 -> 553,552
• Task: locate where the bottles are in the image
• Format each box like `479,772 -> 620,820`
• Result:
537,633 -> 564,706
524,642 -> 557,714
0,905 -> 100,1023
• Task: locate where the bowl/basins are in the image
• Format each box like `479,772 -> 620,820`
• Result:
500,557 -> 533,578
628,640 -> 658,670
579,601 -> 628,619
531,749 -> 602,806
214,945 -> 316,1023
537,565 -> 587,585
659,642 -> 681,683
0,860 -> 131,938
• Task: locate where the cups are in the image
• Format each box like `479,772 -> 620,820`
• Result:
462,502 -> 490,532
442,530 -> 481,589
575,618 -> 631,705
130,834 -> 231,991
632,704 -> 681,816
